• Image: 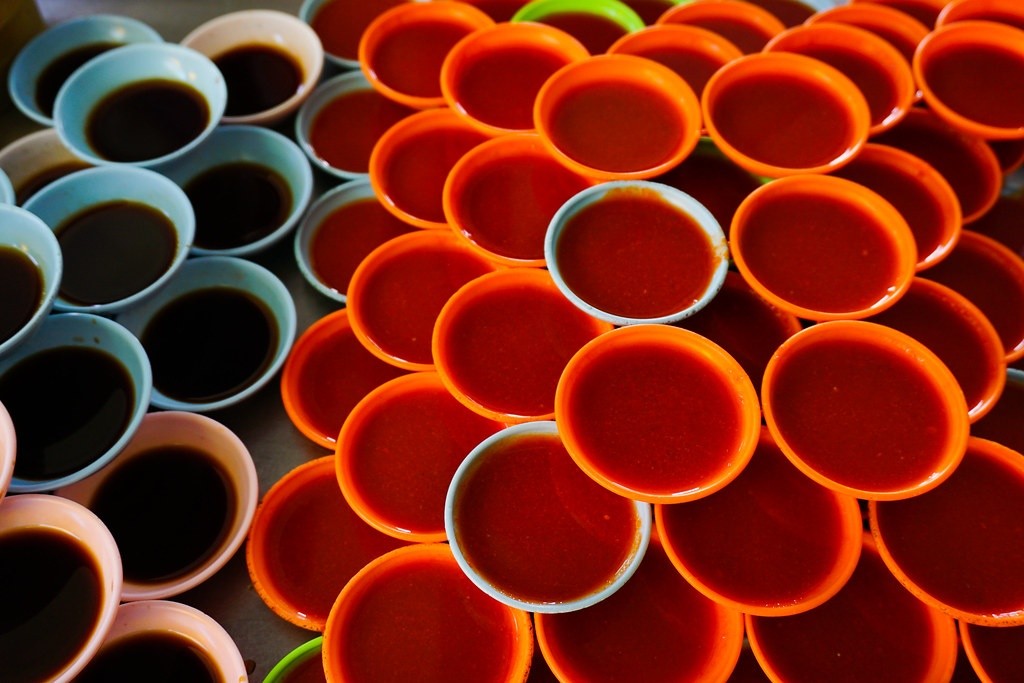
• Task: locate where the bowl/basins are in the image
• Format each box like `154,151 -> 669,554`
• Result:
0,0 -> 1024,683
